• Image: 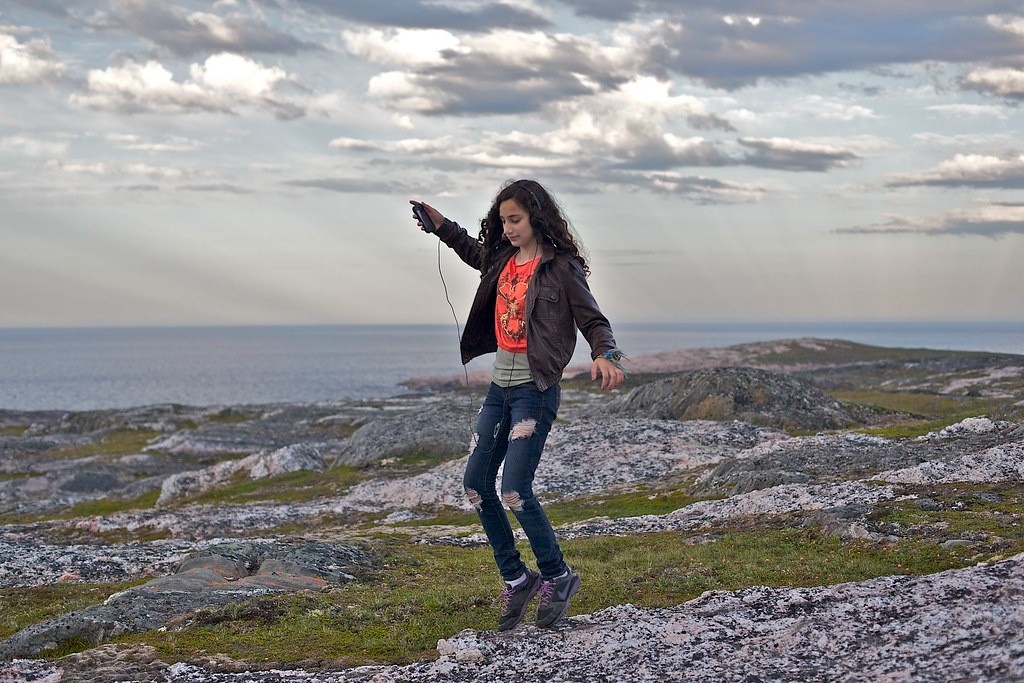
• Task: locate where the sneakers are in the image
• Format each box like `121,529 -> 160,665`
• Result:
498,566 -> 541,631
535,565 -> 580,628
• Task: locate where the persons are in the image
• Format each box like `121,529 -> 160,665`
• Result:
409,179 -> 625,634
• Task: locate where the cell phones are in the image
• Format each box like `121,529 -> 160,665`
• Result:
412,204 -> 436,233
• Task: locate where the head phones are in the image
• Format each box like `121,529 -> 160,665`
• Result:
516,184 -> 543,227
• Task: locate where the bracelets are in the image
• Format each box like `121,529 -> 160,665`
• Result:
595,349 -> 623,364
